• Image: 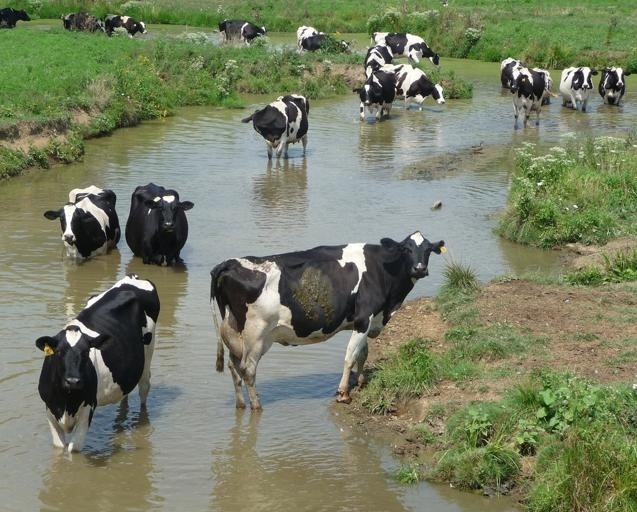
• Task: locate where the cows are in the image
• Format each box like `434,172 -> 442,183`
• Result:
352,33 -> 446,128
43,184 -> 121,261
125,182 -> 194,266
218,19 -> 266,47
35,272 -> 160,452
209,231 -> 445,411
0,7 -> 31,29
60,12 -> 147,40
241,93 -> 309,160
498,55 -> 625,129
295,25 -> 351,56
